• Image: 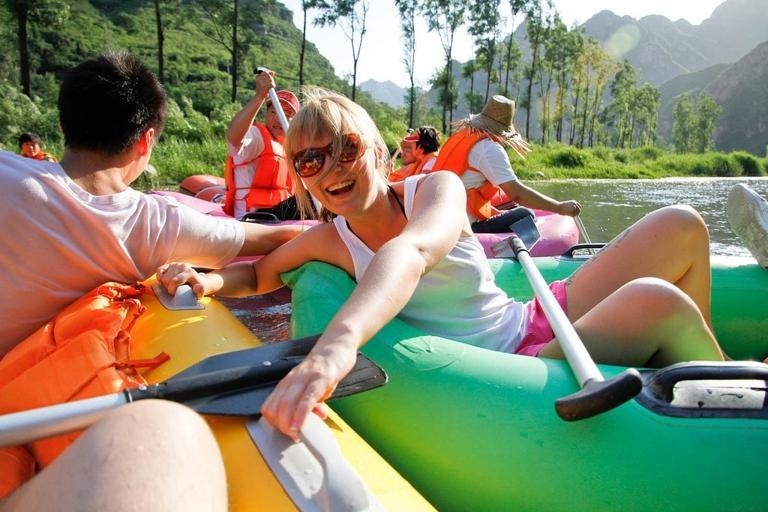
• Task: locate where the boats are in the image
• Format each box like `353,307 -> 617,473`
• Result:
149,188 -> 581,260
277,242 -> 767,512
179,174 -> 229,204
117,264 -> 439,512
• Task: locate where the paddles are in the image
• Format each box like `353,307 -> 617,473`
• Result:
0,333 -> 387,445
490,214 -> 643,422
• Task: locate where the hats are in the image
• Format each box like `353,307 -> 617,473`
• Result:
405,129 -> 421,142
267,89 -> 301,114
450,95 -> 531,160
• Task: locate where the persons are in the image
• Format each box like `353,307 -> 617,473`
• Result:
18,132 -> 56,162
154,94 -> 727,445
0,50 -> 312,360
430,94 -> 582,233
225,68 -> 319,222
406,125 -> 438,174
387,133 -> 417,180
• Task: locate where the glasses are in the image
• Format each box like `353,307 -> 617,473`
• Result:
293,133 -> 377,177
